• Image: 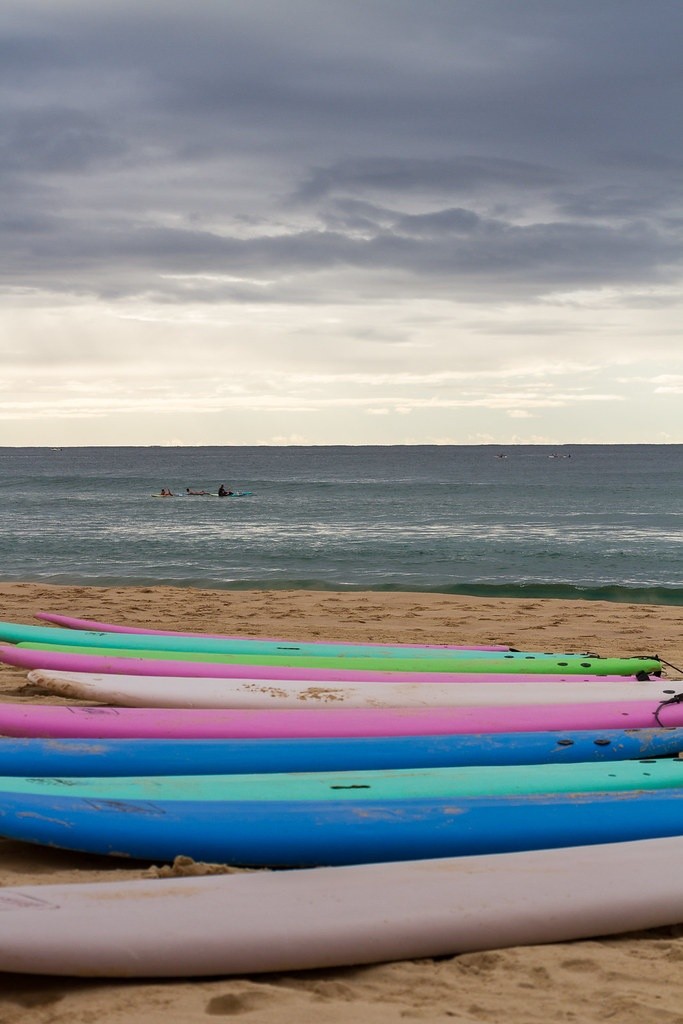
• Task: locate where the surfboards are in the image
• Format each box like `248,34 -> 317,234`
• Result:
0,615 -> 683,978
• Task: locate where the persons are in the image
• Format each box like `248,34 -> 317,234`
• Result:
219,484 -> 227,497
186,488 -> 204,494
160,488 -> 174,496
